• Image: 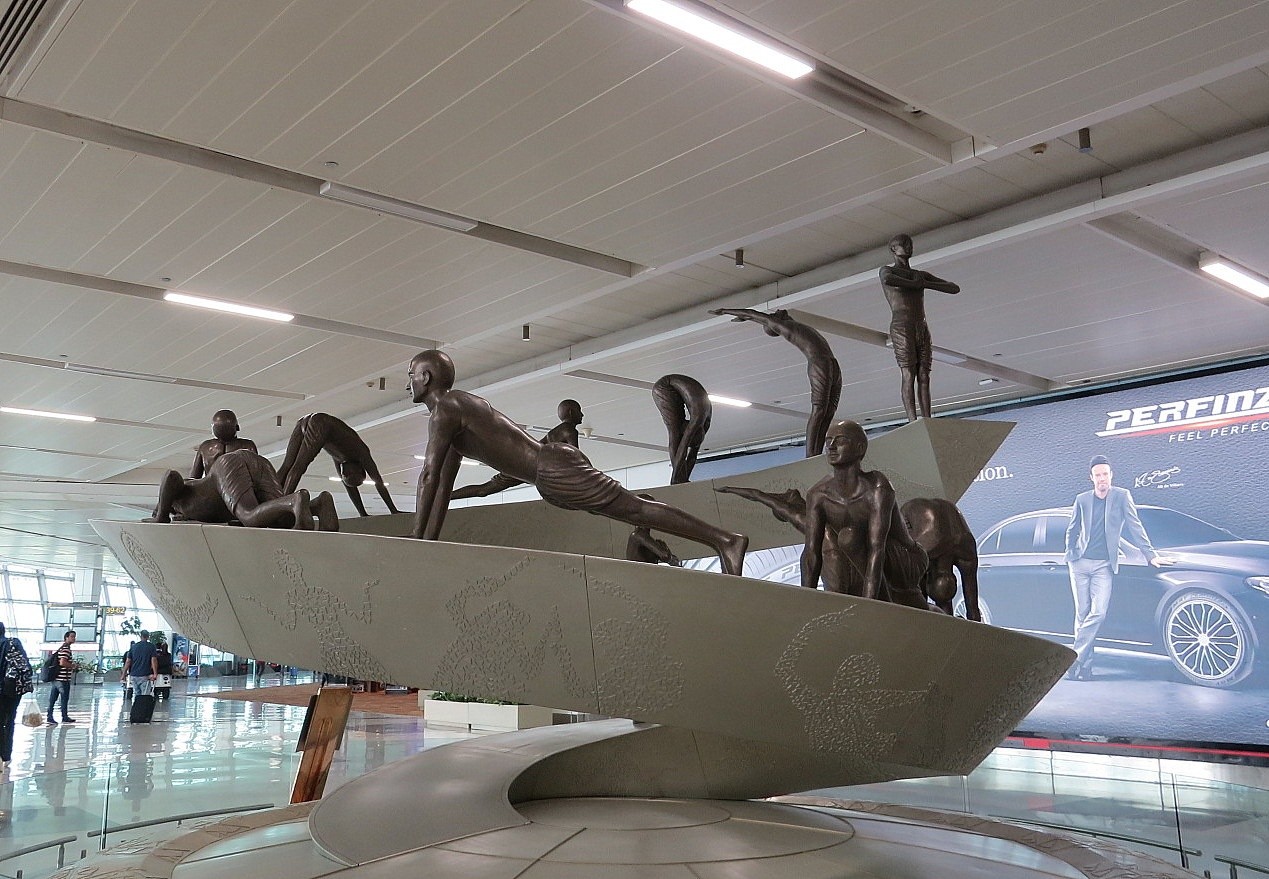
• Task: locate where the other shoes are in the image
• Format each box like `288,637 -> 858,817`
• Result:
46,716 -> 59,724
62,716 -> 76,723
1066,660 -> 1092,681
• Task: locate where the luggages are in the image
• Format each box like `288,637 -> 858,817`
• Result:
129,678 -> 157,724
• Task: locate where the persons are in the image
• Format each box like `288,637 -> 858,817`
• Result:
626,493 -> 679,566
1065,454 -> 1178,682
708,308 -> 842,458
390,350 -> 750,576
47,631 -> 76,724
120,630 -> 158,705
0,622 -> 34,767
879,233 -> 960,421
140,409 -> 340,532
449,398 -> 584,500
652,373 -> 712,484
276,412 -> 412,516
121,640 -> 172,687
713,419 -> 981,622
256,660 -> 265,683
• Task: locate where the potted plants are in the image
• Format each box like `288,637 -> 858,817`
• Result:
32,655 -> 102,685
422,690 -> 553,733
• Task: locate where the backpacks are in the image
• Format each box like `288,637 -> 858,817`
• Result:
4,638 -> 34,696
41,651 -> 61,682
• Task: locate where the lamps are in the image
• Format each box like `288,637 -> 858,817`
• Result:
0,0 -> 1269,438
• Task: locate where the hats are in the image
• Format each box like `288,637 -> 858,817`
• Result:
1091,455 -> 1112,469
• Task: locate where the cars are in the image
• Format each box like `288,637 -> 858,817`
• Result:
952,505 -> 1269,687
